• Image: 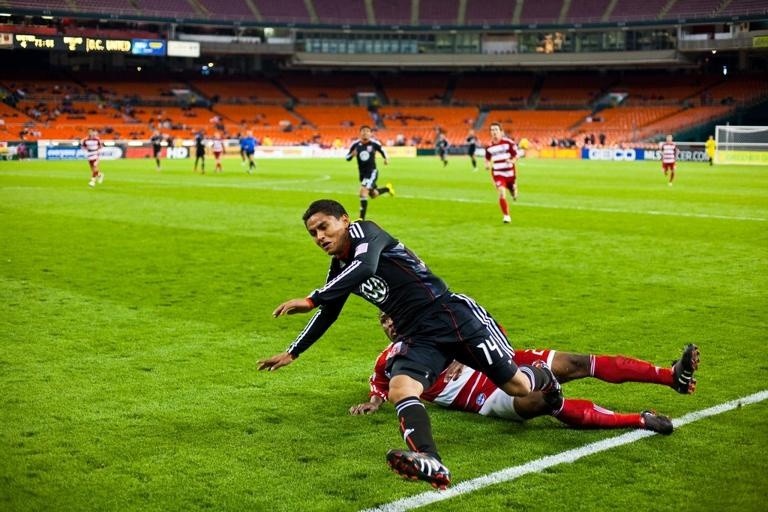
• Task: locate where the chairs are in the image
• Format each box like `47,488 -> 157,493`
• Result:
0,73 -> 768,149
0,0 -> 768,41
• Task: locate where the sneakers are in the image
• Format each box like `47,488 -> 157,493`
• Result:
503,214 -> 511,223
641,408 -> 673,436
386,449 -> 450,491
533,360 -> 564,415
88,172 -> 104,186
672,342 -> 699,395
386,183 -> 395,197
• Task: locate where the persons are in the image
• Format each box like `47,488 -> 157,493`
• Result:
346,126 -> 395,221
255,199 -> 562,490
1,83 -> 734,187
348,310 -> 700,435
484,123 -> 519,224
